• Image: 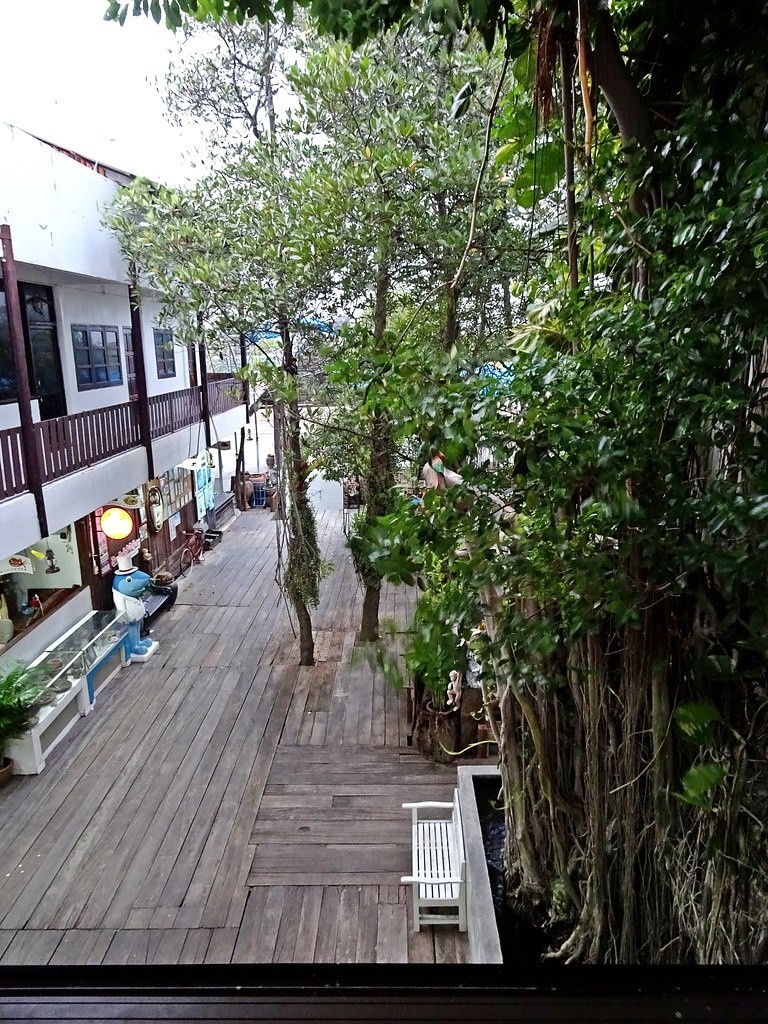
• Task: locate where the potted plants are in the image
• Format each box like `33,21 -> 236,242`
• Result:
0,659 -> 56,788
403,593 -> 483,766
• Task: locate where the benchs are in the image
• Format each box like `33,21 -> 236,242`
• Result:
402,787 -> 468,933
131,579 -> 172,635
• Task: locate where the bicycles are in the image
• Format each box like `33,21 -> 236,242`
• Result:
180,530 -> 205,578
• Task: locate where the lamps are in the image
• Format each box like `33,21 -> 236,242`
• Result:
25,284 -> 54,314
